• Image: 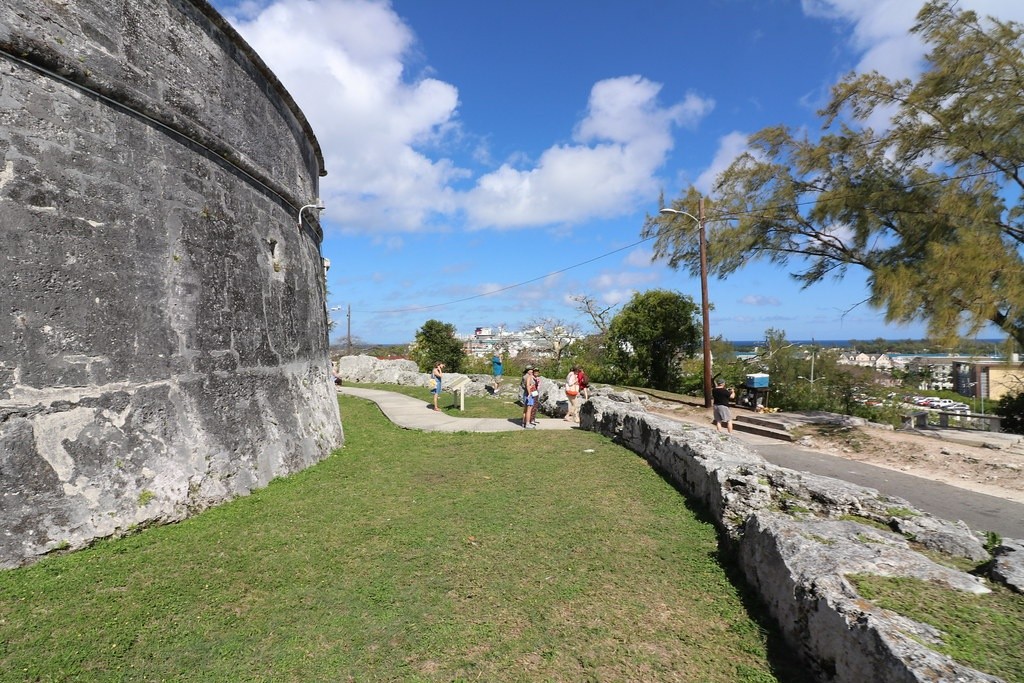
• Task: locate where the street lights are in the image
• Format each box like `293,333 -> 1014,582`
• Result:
796,352 -> 826,394
659,199 -> 713,409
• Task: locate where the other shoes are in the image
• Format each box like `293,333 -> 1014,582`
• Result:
434,408 -> 440,411
494,389 -> 500,393
575,419 -> 580,423
522,423 -> 535,429
565,416 -> 571,421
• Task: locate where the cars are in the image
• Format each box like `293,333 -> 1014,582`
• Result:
851,391 -> 971,413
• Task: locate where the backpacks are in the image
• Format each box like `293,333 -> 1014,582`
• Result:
581,374 -> 590,388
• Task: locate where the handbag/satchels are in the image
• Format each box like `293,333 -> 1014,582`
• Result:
531,390 -> 538,397
429,379 -> 437,391
566,384 -> 579,396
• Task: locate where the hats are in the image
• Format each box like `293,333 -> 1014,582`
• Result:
718,379 -> 726,383
526,365 -> 532,369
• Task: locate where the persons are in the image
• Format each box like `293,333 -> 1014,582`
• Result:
432,362 -> 444,411
332,363 -> 342,389
565,366 -> 589,422
712,379 -> 734,434
521,365 -> 539,428
493,352 -> 502,393
